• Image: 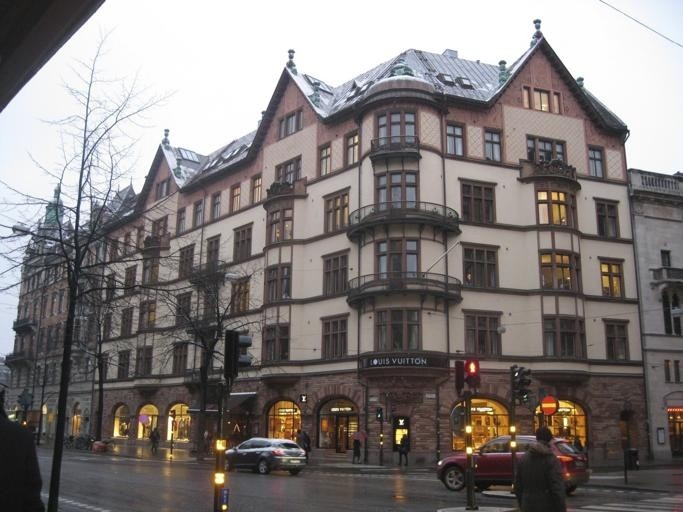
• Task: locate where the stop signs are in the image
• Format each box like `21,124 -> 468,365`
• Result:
542,396 -> 559,416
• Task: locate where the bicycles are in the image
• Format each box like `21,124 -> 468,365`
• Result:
149,437 -> 159,455
64,433 -> 95,450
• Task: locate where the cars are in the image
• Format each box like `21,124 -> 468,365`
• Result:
225,438 -> 307,475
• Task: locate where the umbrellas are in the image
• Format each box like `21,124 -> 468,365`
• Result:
351,431 -> 368,440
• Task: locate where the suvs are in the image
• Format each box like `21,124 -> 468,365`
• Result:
437,435 -> 590,493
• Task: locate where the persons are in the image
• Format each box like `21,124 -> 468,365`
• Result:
149,427 -> 160,455
513,425 -> 567,512
398,434 -> 410,467
0,388 -> 45,512
303,431 -> 311,464
295,429 -> 304,448
353,439 -> 361,465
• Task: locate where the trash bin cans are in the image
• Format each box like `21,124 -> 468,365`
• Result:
627,448 -> 640,471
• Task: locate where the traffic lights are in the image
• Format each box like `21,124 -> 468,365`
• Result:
376,408 -> 382,421
465,361 -> 479,376
513,366 -> 531,392
236,336 -> 253,367
